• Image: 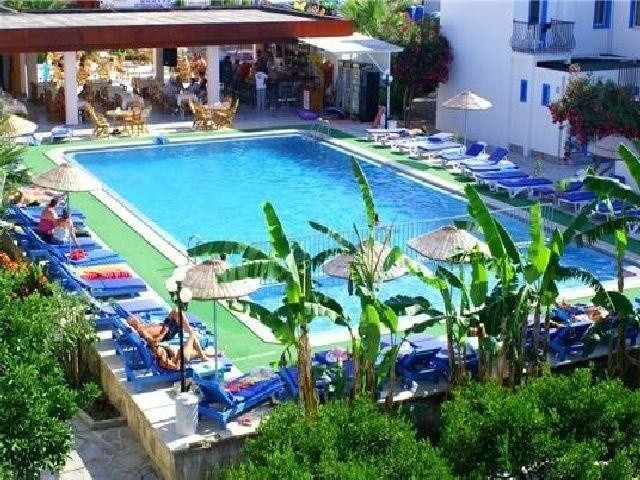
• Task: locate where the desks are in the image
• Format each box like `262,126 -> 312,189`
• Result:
2,91 -> 27,119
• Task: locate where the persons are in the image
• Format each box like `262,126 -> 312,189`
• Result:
186,78 -> 208,105
219,45 -> 300,112
556,298 -> 607,320
38,196 -> 60,234
127,307 -> 202,343
152,332 -> 226,372
50,208 -> 78,248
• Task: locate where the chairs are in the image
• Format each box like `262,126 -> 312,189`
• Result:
192,372 -> 284,426
284,299 -> 640,401
2,187 -> 231,389
366,122 -> 640,241
34,80 -> 240,141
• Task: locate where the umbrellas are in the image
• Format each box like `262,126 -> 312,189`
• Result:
182,259 -> 263,378
319,238 -> 416,284
406,225 -> 494,264
0,113 -> 41,151
441,89 -> 493,148
33,161 -> 101,252
587,132 -> 639,161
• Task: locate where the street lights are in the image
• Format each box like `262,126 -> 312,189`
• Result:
166,266 -> 193,392
382,69 -> 393,128
